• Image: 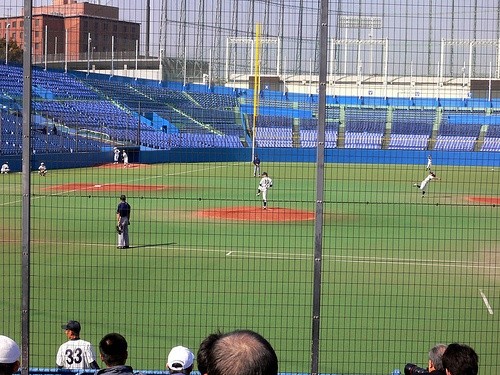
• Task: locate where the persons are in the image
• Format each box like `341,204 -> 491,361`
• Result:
52,124 -> 57,136
425,154 -> 432,171
115,195 -> 131,249
427,343 -> 479,375
94,332 -> 136,375
56,320 -> 100,375
1,161 -> 10,175
197,328 -> 278,375
166,345 -> 195,375
0,335 -> 21,375
121,150 -> 131,168
38,161 -> 47,177
256,171 -> 273,210
253,154 -> 260,178
413,171 -> 442,198
41,126 -> 48,135
112,145 -> 121,165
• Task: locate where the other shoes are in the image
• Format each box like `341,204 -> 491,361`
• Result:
124,246 -> 129,248
117,246 -> 124,249
264,207 -> 266,210
256,193 -> 259,196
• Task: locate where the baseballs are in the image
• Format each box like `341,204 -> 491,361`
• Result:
492,169 -> 494,170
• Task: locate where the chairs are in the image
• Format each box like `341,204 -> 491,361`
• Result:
0,64 -> 500,158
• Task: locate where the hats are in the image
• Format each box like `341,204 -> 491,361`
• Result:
168,345 -> 195,371
62,321 -> 81,332
0,335 -> 20,363
261,172 -> 267,175
120,195 -> 126,200
